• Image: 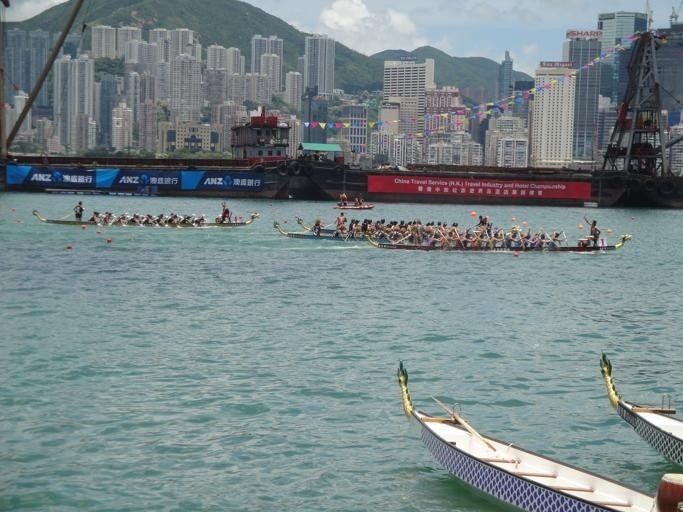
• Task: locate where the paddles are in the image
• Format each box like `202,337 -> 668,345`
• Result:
431,396 -> 497,452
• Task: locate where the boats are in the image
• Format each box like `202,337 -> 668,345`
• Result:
397,360 -> 660,512
32,210 -> 261,227
332,203 -> 375,209
364,233 -> 632,251
599,352 -> 683,467
273,213 -> 475,240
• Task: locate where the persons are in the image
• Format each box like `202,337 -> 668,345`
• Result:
311,210 -> 602,248
73,200 -> 232,226
339,192 -> 365,206
